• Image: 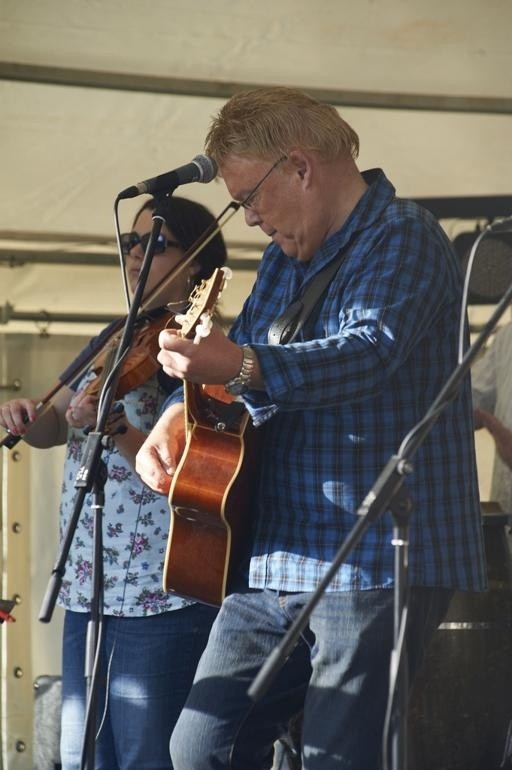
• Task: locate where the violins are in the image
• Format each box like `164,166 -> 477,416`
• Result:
83,308 -> 188,402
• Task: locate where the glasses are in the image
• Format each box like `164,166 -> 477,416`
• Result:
242,155 -> 287,209
119,231 -> 182,256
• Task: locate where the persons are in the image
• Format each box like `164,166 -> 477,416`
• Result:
0,194 -> 229,770
132,83 -> 490,769
464,319 -> 512,532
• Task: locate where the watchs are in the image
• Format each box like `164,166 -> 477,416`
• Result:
224,345 -> 254,395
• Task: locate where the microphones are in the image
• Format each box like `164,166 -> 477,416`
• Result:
119,153 -> 219,199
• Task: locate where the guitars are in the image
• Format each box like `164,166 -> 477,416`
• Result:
160,267 -> 260,609
